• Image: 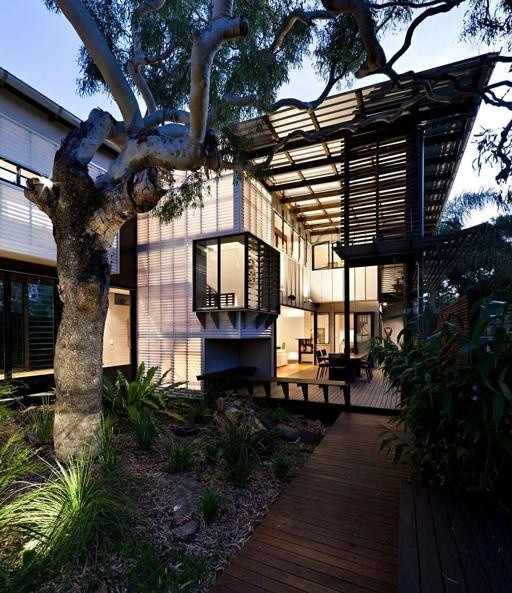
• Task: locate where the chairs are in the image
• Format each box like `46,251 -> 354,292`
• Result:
360,351 -> 374,382
316,349 -> 330,379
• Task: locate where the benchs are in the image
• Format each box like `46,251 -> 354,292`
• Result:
243,375 -> 350,405
197,365 -> 258,392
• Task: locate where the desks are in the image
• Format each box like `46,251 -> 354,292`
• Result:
322,351 -> 368,383
